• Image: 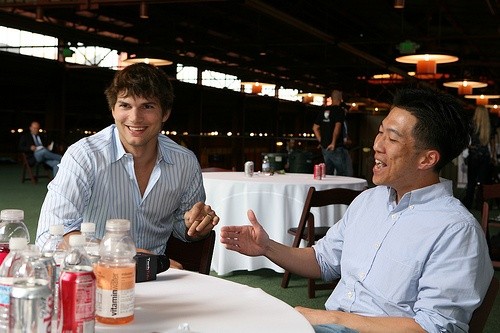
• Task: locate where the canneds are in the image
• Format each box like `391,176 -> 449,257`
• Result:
245,161 -> 254,177
9,278 -> 54,333
319,163 -> 326,178
58,266 -> 96,333
314,165 -> 322,180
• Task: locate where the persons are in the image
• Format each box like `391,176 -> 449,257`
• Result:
280,137 -> 295,173
464,104 -> 494,210
339,100 -> 354,176
220,88 -> 494,333
34,62 -> 219,270
313,89 -> 345,177
21,121 -> 62,177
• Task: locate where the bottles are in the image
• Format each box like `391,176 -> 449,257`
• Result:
60,235 -> 92,274
0,209 -> 30,266
9,244 -> 50,333
262,156 -> 270,176
80,223 -> 101,266
0,238 -> 28,333
93,219 -> 137,324
41,225 -> 73,320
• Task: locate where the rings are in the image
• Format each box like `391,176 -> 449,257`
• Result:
207,213 -> 212,218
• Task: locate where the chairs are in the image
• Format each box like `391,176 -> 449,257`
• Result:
281,186 -> 371,298
22,152 -> 52,184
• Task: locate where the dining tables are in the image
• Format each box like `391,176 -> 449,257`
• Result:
202,172 -> 369,275
95,268 -> 316,333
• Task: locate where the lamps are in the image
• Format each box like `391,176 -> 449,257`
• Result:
393,39 -> 500,110
121,1 -> 173,67
